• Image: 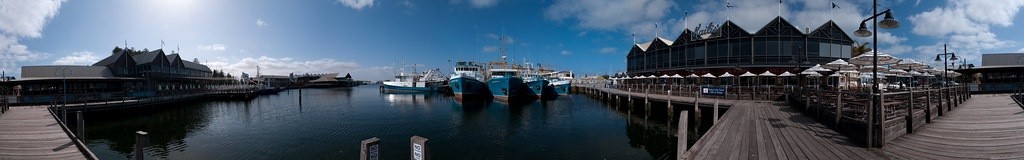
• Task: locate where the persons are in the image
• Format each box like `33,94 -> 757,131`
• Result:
898,80 -> 902,89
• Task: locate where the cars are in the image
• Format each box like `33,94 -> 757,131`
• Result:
888,82 -> 906,92
906,80 -> 919,87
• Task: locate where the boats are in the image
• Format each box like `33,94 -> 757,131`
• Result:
377,25 -> 577,108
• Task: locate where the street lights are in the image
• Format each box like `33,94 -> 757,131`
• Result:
853,0 -> 900,124
54,66 -> 74,105
934,44 -> 958,99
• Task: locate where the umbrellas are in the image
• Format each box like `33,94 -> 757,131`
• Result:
610,50 -> 983,89
